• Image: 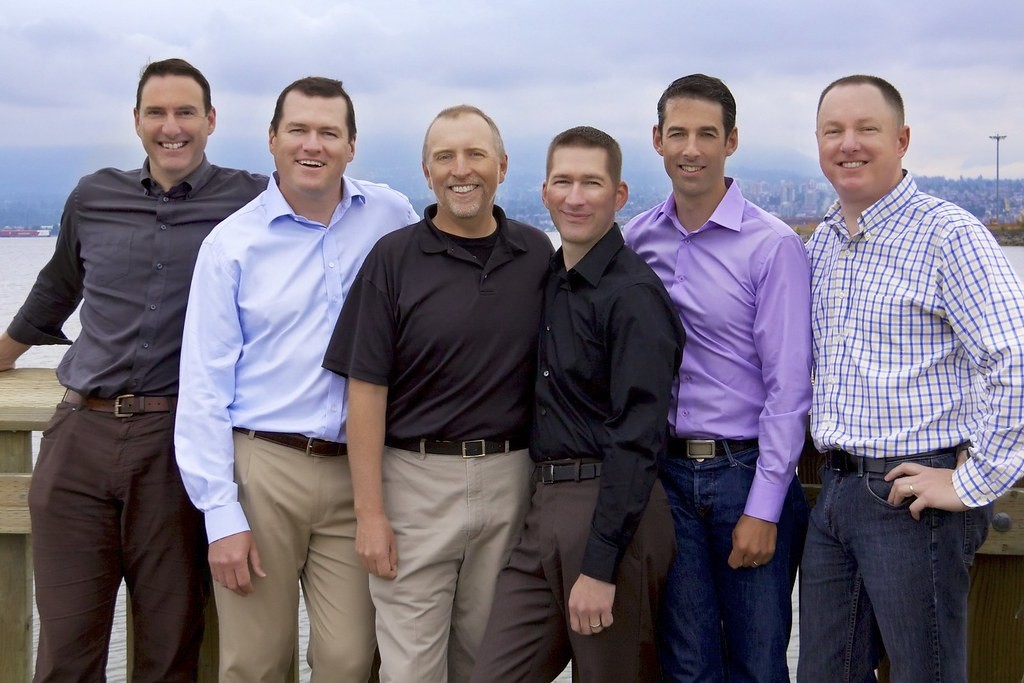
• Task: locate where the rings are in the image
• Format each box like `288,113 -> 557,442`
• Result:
752,561 -> 759,567
590,623 -> 601,628
909,485 -> 914,494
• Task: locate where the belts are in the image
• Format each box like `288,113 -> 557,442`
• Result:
536,463 -> 603,484
64,387 -> 180,417
234,426 -> 349,457
671,438 -> 758,459
384,437 -> 531,458
822,446 -> 959,473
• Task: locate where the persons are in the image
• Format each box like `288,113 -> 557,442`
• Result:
0,59 -> 270,683
174,77 -> 421,683
475,125 -> 685,683
625,76 -> 813,683
805,76 -> 1024,683
322,106 -> 556,683
